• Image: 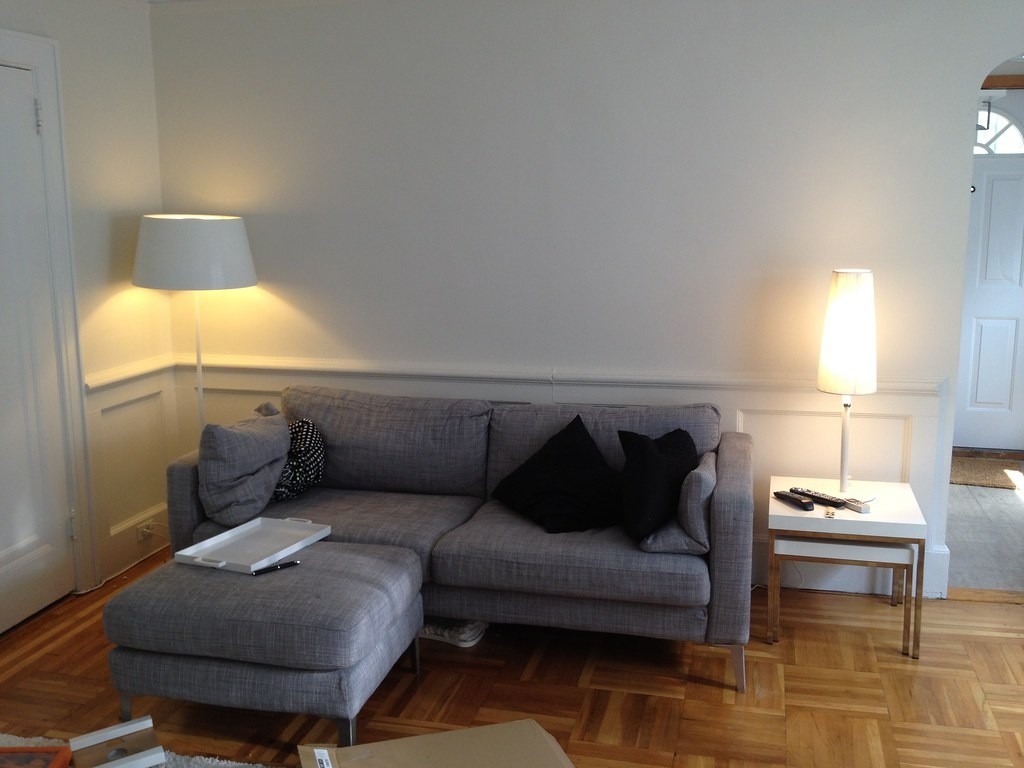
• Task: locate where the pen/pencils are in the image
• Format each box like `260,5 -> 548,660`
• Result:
252,560 -> 300,576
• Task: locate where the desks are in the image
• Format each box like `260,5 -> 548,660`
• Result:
766,476 -> 928,659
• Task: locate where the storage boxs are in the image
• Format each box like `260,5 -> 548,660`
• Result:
298,718 -> 576,767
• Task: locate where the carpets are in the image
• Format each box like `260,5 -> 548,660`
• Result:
949,456 -> 1020,490
0,733 -> 269,768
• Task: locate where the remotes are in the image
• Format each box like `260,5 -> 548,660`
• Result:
773,487 -> 870,514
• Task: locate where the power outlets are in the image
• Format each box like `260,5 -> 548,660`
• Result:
137,519 -> 154,543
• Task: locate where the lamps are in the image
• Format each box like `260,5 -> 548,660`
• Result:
132,212 -> 258,434
817,269 -> 877,492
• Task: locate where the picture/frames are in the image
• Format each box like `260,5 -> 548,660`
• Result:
0,746 -> 73,768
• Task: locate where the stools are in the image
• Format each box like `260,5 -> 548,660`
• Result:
102,543 -> 424,747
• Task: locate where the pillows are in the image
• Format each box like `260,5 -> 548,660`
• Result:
640,452 -> 716,554
200,401 -> 290,527
492,414 -> 616,535
614,428 -> 701,541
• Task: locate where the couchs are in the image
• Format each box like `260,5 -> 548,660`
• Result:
165,384 -> 753,693
